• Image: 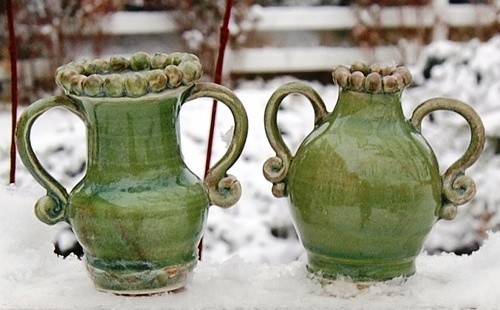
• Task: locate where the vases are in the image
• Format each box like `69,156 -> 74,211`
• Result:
16,53 -> 248,297
264,62 -> 484,297
